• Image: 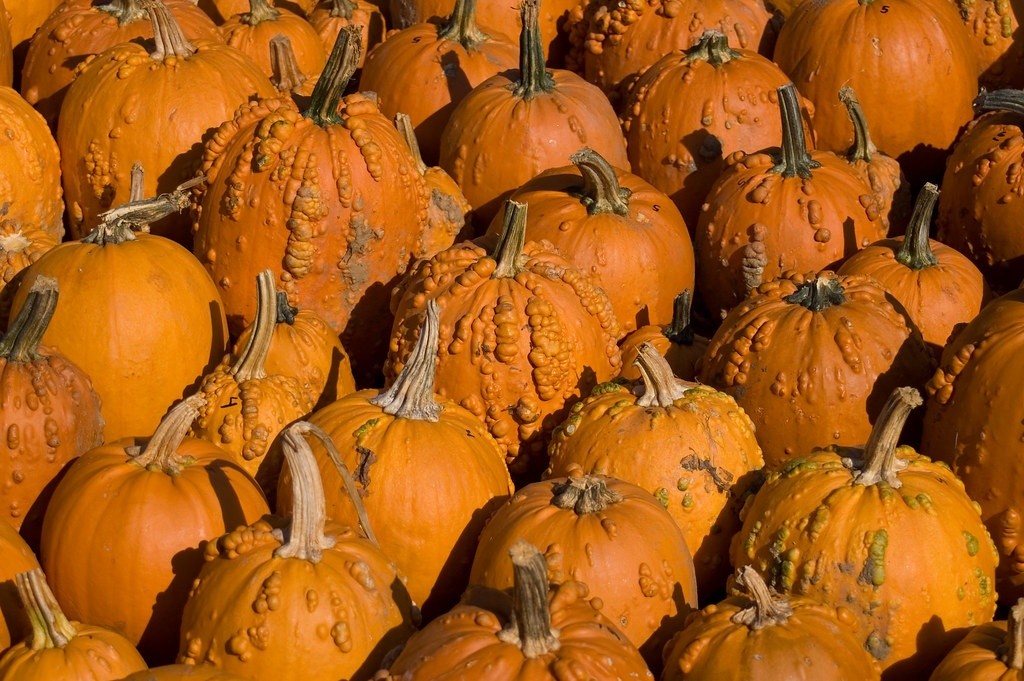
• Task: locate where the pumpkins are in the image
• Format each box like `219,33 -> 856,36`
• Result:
0,0 -> 1024,681
